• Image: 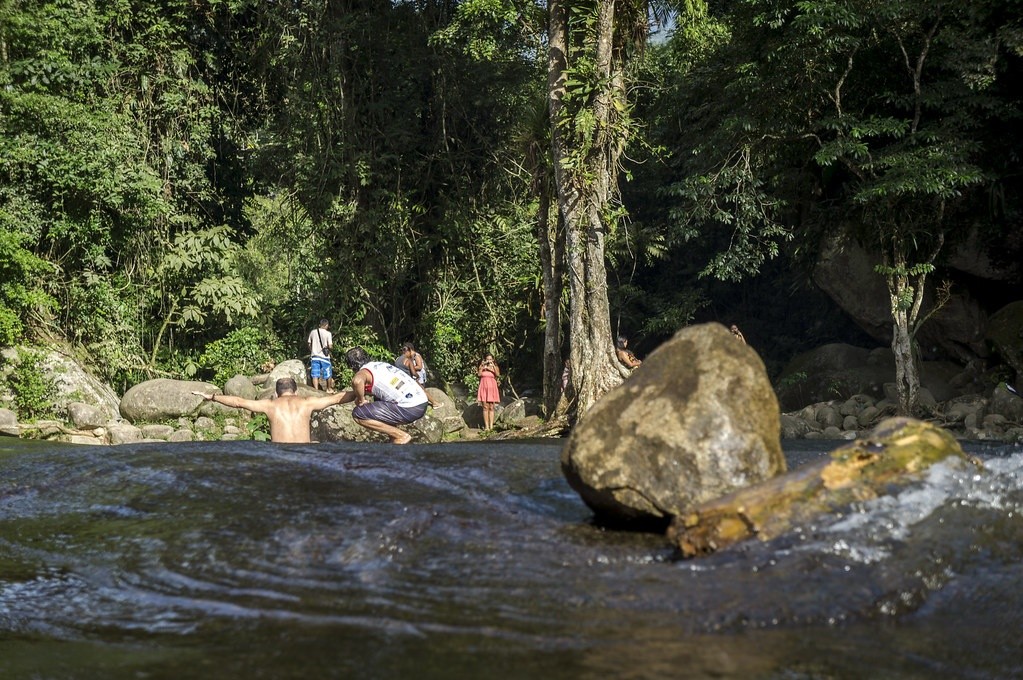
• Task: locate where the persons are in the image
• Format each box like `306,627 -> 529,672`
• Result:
559,358 -> 571,391
395,345 -> 444,409
478,353 -> 500,431
346,347 -> 429,444
730,323 -> 746,344
405,342 -> 427,386
308,319 -> 337,394
191,377 -> 371,443
615,335 -> 641,372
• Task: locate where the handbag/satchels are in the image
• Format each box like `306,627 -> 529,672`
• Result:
322,347 -> 329,356
